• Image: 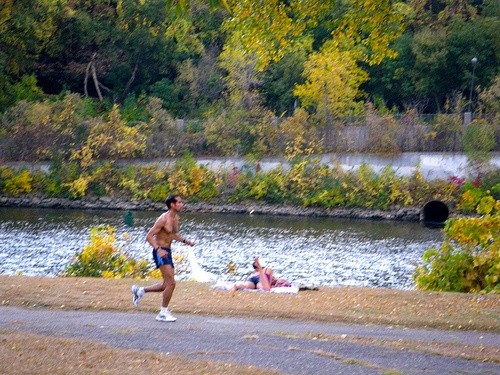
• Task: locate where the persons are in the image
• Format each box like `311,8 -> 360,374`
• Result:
229,255 -> 272,297
131,194 -> 196,322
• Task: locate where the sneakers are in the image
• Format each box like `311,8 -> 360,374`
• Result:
132,285 -> 142,310
156,310 -> 177,322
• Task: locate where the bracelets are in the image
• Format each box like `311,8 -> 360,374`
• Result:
183,238 -> 186,243
